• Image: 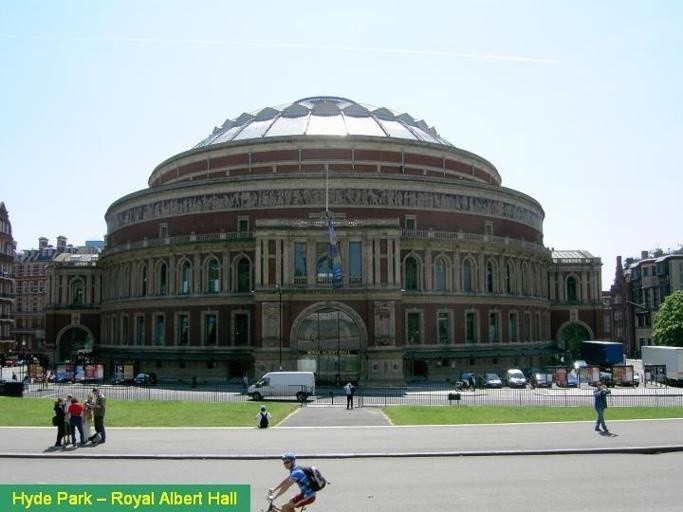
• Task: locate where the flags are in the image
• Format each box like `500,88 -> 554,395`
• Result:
328,222 -> 344,288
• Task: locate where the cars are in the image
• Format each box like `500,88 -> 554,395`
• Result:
4,356 -> 70,384
528,367 -> 640,388
480,372 -> 502,388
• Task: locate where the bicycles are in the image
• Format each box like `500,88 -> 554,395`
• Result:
261,491 -> 306,512
454,378 -> 474,393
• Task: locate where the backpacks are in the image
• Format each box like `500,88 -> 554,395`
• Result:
259,412 -> 268,429
295,463 -> 326,492
89,433 -> 104,446
53,416 -> 58,427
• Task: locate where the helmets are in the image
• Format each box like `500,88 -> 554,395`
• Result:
281,451 -> 296,461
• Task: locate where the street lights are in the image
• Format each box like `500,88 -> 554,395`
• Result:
21,339 -> 26,359
274,281 -> 283,370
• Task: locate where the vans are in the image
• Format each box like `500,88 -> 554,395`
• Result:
504,368 -> 527,389
245,370 -> 317,403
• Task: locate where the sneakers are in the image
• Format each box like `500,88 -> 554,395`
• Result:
594,428 -> 609,433
55,441 -> 92,450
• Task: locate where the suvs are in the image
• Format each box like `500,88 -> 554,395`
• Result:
460,371 -> 476,387
132,372 -> 156,386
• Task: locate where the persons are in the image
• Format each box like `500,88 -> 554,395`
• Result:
593,382 -> 611,433
54,388 -> 105,448
254,405 -> 271,428
344,382 -> 354,410
268,452 -> 316,512
458,377 -> 466,386
467,375 -> 475,391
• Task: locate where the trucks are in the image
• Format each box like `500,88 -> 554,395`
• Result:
640,345 -> 682,388
